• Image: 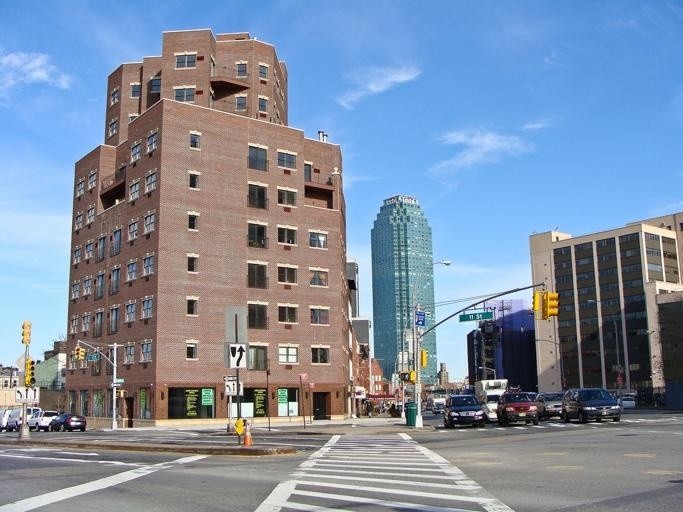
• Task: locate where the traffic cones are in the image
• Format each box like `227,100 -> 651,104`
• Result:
242,422 -> 253,447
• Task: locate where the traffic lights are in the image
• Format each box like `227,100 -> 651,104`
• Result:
420,349 -> 428,368
79,348 -> 85,360
74,347 -> 80,360
409,370 -> 416,381
119,389 -> 127,397
400,373 -> 409,381
531,292 -> 539,312
542,291 -> 560,320
25,357 -> 35,386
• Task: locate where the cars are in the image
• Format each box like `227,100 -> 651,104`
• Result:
26,410 -> 59,432
535,392 -> 564,421
561,387 -> 619,424
421,378 -> 647,415
47,413 -> 85,431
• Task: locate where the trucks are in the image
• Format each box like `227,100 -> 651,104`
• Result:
473,378 -> 508,424
0,409 -> 12,432
6,406 -> 41,431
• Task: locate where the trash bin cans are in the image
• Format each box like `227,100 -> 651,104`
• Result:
405,401 -> 418,426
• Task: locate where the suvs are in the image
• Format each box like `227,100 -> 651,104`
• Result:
496,391 -> 538,427
443,394 -> 485,428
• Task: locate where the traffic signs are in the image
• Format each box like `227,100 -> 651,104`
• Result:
458,312 -> 493,322
20,320 -> 31,344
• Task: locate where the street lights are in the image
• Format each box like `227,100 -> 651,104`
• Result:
409,261 -> 451,404
478,366 -> 496,380
534,339 -> 566,392
587,299 -> 622,389
